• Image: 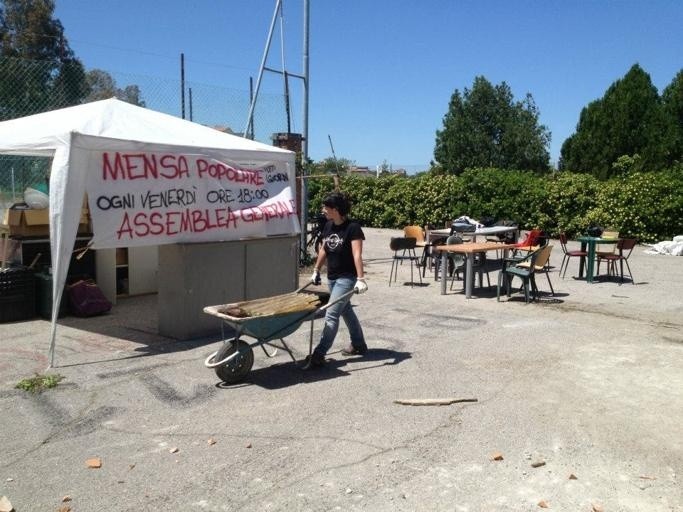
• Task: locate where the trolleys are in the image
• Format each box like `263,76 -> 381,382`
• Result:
199,278 -> 367,385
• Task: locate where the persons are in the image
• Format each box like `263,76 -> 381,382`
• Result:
298,192 -> 372,370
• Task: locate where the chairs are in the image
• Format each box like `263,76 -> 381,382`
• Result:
389,224 -> 642,305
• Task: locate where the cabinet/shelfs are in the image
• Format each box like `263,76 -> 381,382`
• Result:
94,245 -> 158,307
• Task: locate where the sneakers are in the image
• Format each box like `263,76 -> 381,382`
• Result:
299,355 -> 325,370
342,344 -> 368,355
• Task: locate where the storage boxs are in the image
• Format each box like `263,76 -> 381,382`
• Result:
2,201 -> 88,239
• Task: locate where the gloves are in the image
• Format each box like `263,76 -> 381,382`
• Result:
311,270 -> 320,285
354,278 -> 367,294
